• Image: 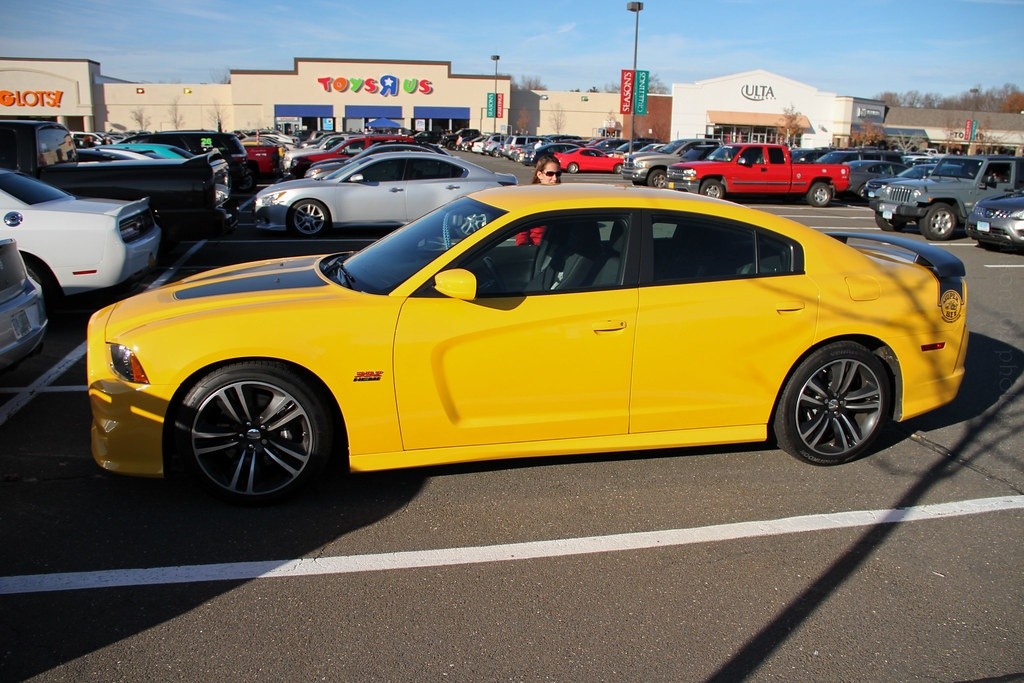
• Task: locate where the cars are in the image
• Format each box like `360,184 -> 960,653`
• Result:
788,145 -> 976,202
0,239 -> 49,373
0,120 -> 724,240
963,188 -> 1024,253
254,151 -> 518,244
0,167 -> 162,314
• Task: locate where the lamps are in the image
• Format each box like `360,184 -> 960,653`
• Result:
540,95 -> 548,100
818,124 -> 824,130
581,96 -> 589,101
137,88 -> 145,94
184,88 -> 193,94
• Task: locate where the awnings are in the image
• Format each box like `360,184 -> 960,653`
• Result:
704,110 -> 810,128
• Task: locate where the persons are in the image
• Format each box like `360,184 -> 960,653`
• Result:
83,135 -> 94,148
456,134 -> 463,149
517,155 -> 564,246
364,127 -> 393,134
101,137 -> 113,145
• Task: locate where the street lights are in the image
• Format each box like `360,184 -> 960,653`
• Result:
970,89 -> 979,122
488,55 -> 500,136
626,0 -> 644,155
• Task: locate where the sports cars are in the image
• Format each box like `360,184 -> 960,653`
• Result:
85,184 -> 969,527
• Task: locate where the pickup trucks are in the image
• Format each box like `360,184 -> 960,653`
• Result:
665,142 -> 851,208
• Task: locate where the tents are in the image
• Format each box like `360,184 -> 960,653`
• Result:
366,117 -> 401,134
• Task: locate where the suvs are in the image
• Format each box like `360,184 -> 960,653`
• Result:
869,156 -> 1024,242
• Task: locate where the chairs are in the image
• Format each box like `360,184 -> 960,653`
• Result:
523,222 -> 601,294
591,217 -> 630,288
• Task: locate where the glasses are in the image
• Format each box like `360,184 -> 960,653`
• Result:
541,171 -> 562,177
